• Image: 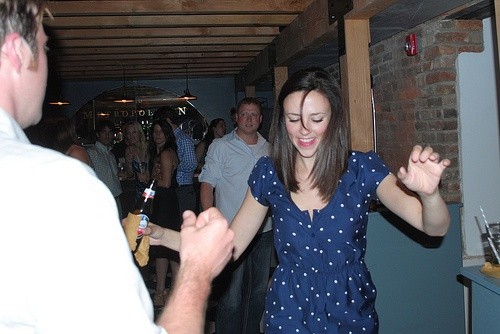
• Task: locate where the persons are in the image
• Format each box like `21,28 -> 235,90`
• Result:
0,0 -> 234,334
121,67 -> 450,334
42,97 -> 273,334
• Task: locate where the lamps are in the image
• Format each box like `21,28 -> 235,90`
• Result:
113,65 -> 134,103
176,63 -> 197,100
48,77 -> 70,106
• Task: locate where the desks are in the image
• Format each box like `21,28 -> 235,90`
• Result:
461,262 -> 500,334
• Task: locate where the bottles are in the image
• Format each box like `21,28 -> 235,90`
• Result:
124,179 -> 157,253
111,129 -> 124,144
141,119 -> 152,143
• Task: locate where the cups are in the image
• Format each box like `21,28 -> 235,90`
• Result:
486,222 -> 500,266
118,162 -> 125,174
